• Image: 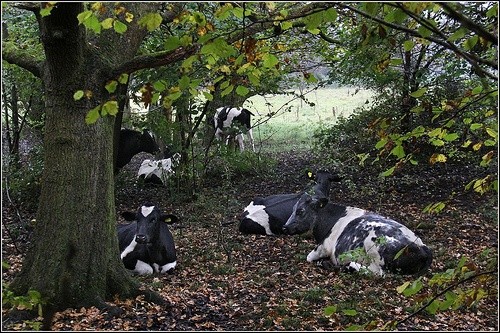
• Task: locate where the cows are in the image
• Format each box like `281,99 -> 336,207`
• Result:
214,107 -> 255,153
118,128 -> 159,168
281,191 -> 433,278
237,170 -> 343,237
116,202 -> 179,277
